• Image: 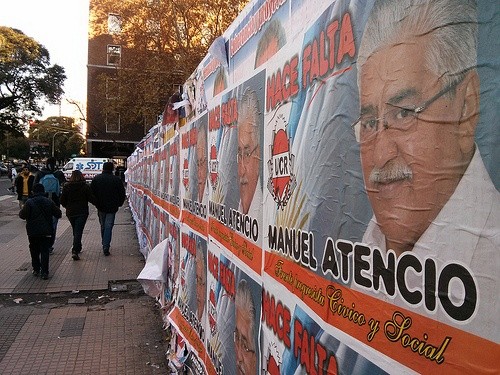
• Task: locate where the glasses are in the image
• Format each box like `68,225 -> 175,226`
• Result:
196,278 -> 205,288
196,157 -> 206,171
233,331 -> 254,354
351,72 -> 467,142
236,145 -> 258,163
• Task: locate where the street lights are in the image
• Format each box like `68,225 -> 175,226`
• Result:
52,131 -> 70,158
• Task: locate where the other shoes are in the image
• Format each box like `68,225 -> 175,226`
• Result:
48,247 -> 54,255
103,248 -> 110,256
32,269 -> 40,277
72,253 -> 80,260
39,271 -> 48,279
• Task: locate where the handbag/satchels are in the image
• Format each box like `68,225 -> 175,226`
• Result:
45,192 -> 58,199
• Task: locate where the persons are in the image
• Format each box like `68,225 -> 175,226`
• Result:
6,157 -> 126,280
348,0 -> 500,347
192,22 -> 287,375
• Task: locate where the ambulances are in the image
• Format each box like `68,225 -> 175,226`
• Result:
61,158 -> 115,185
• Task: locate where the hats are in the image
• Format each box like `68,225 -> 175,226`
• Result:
103,162 -> 113,170
33,184 -> 44,193
